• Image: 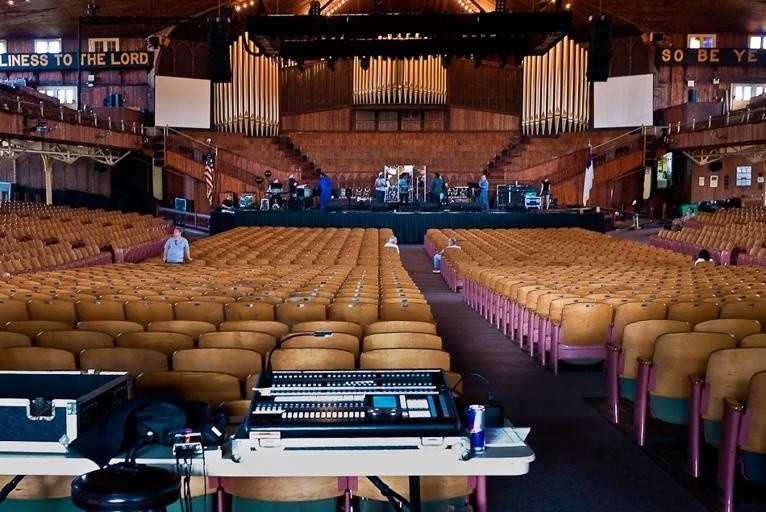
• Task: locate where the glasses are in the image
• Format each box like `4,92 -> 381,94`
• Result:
175,240 -> 177,245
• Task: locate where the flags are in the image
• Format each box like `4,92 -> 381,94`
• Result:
583,148 -> 594,207
204,144 -> 214,207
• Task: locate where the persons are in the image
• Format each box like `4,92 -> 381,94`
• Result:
399,173 -> 410,204
694,250 -> 711,266
540,177 -> 552,212
430,172 -> 442,210
384,236 -> 400,254
374,172 -> 387,203
287,171 -> 298,200
221,194 -> 234,208
315,173 -> 331,209
477,174 -> 490,211
432,238 -> 456,273
684,207 -> 695,222
268,178 -> 284,205
162,225 -> 193,266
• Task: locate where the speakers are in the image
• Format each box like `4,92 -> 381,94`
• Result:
325,200 -> 343,212
507,202 -> 526,212
369,200 -> 388,211
420,201 -> 438,211
464,204 -> 481,212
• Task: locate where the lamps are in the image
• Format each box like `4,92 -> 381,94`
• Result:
36,83 -> 75,106
689,36 -> 700,49
748,33 -> 761,51
733,84 -> 766,102
33,38 -> 61,54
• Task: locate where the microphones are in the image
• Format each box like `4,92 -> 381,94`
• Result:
264,329 -> 333,375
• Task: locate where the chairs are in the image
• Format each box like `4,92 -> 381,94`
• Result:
421,204 -> 765,509
0,204 -> 478,510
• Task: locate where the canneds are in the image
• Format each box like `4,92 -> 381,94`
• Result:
468,404 -> 487,454
